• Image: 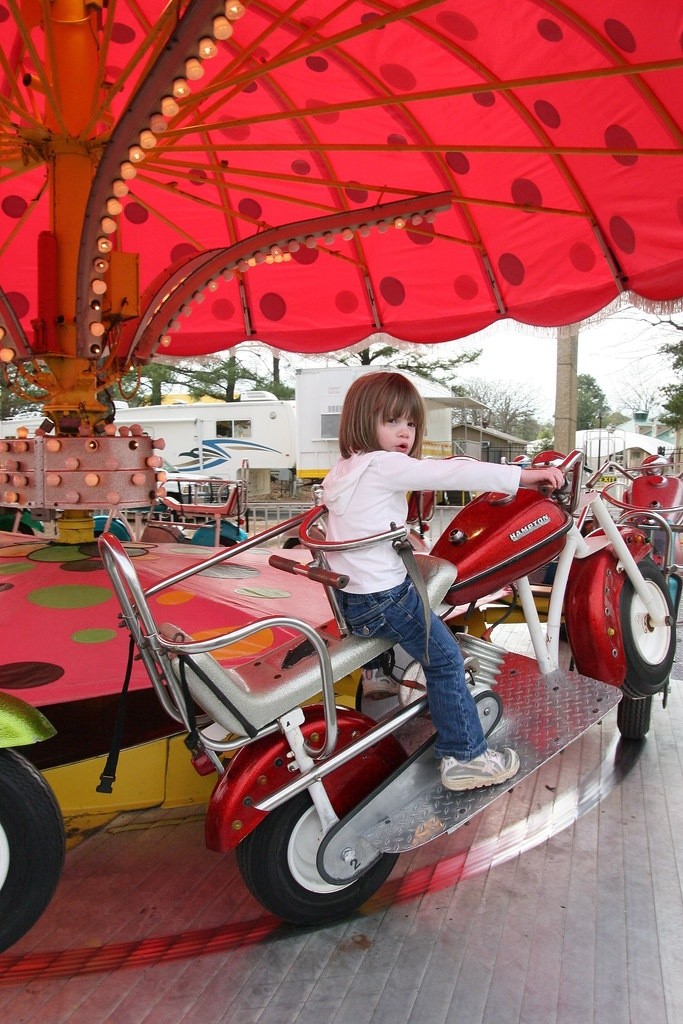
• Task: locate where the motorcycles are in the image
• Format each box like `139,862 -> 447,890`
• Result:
0,692 -> 66,951
96,448 -> 683,927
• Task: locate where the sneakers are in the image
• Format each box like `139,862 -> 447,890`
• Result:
441,746 -> 519,790
362,667 -> 399,701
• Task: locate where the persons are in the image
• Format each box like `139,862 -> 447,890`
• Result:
319,372 -> 564,789
